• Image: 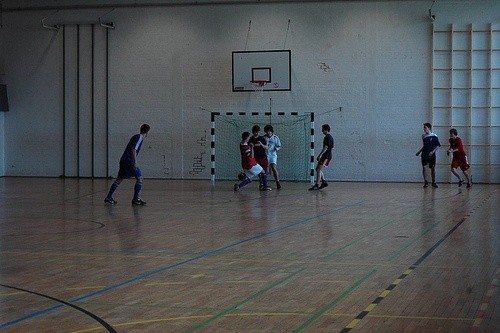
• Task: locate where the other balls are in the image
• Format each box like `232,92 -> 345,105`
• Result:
239,173 -> 246,181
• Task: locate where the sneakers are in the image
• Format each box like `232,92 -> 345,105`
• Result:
103,197 -> 118,204
465,182 -> 472,188
318,180 -> 328,189
308,183 -> 319,191
132,199 -> 147,206
458,178 -> 464,189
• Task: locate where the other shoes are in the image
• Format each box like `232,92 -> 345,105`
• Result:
276,184 -> 281,189
431,183 -> 438,188
233,184 -> 239,191
423,180 -> 428,188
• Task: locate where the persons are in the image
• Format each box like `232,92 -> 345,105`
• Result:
416,123 -> 441,187
447,128 -> 472,188
308,124 -> 334,191
104,124 -> 150,206
233,124 -> 282,192
